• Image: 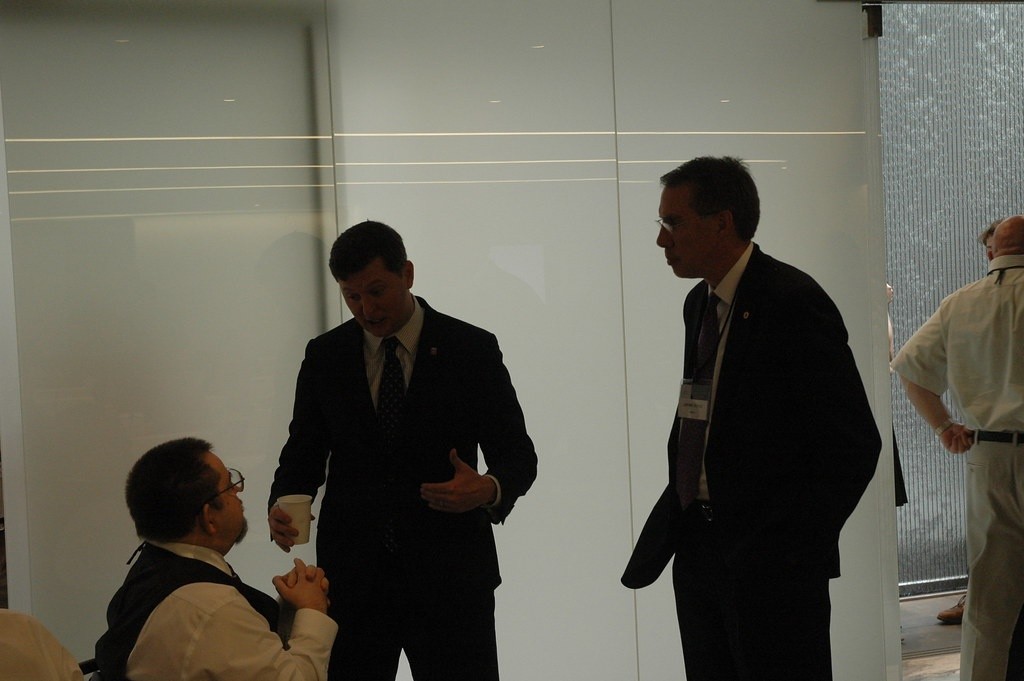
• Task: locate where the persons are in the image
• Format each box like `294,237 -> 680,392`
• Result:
936,218 -> 1024,679
892,214 -> 1024,680
622,156 -> 885,681
0,608 -> 85,681
93,438 -> 341,681
264,220 -> 538,680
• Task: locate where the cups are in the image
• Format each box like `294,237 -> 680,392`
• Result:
277,494 -> 311,545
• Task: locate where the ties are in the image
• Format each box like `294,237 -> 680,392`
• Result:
673,292 -> 722,511
377,337 -> 407,554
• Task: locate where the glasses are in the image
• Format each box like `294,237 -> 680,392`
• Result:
655,205 -> 731,234
196,467 -> 245,515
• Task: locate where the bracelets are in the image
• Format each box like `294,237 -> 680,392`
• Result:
935,418 -> 954,435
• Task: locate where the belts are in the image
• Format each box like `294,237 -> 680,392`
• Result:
967,429 -> 1023,445
689,498 -> 717,523
